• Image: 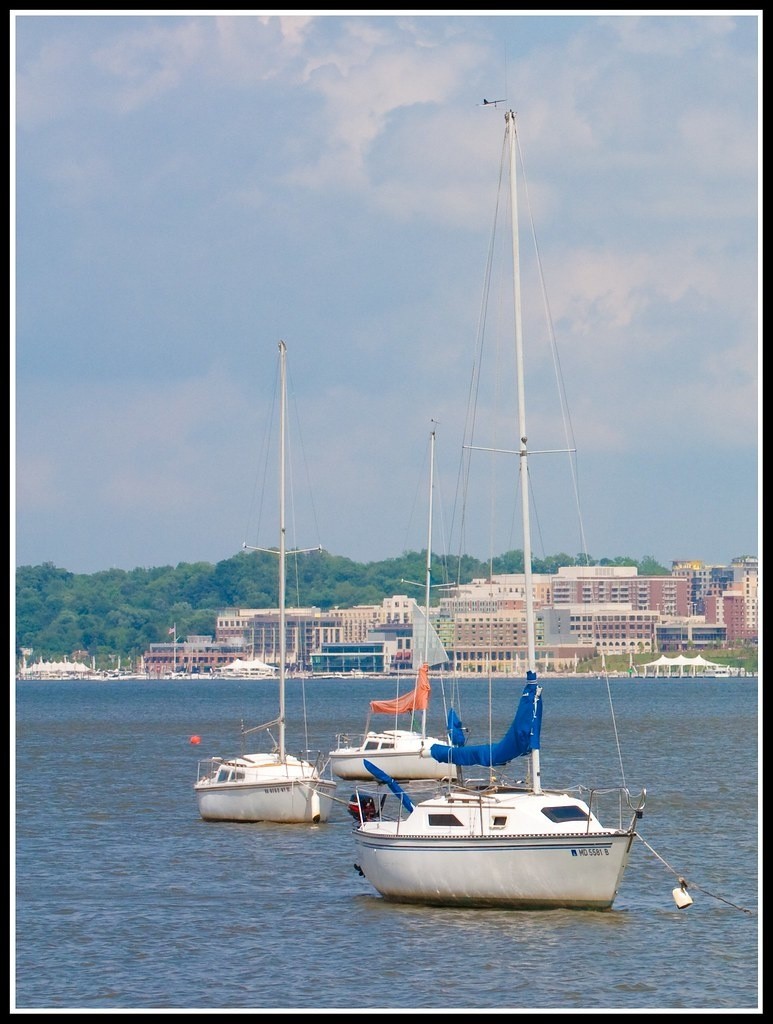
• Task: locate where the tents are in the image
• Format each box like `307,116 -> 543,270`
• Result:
214,658 -> 281,678
32,660 -> 91,679
639,654 -> 726,678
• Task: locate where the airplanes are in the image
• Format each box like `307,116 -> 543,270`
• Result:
479,98 -> 508,107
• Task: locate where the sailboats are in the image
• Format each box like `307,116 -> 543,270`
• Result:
349,106 -> 651,910
326,418 -> 461,782
191,336 -> 338,825
